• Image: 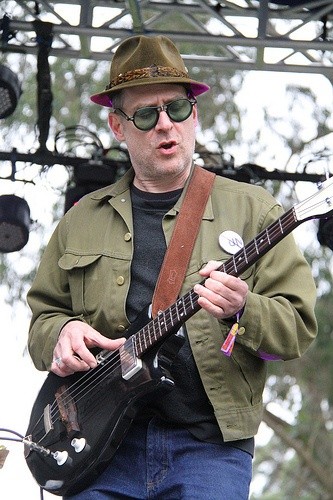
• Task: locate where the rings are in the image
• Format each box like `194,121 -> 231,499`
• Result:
55,357 -> 63,366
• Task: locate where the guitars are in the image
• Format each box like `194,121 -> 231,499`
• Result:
24,178 -> 333,494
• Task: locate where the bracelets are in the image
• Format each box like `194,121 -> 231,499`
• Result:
220,304 -> 245,357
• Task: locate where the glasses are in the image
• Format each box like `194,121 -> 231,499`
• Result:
114,91 -> 197,131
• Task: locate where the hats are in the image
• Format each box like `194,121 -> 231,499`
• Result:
89,35 -> 210,108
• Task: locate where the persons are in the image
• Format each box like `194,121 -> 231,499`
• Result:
27,36 -> 318,500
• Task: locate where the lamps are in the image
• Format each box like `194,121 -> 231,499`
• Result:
0,64 -> 21,120
317,215 -> 333,252
0,194 -> 30,254
63,164 -> 117,216
